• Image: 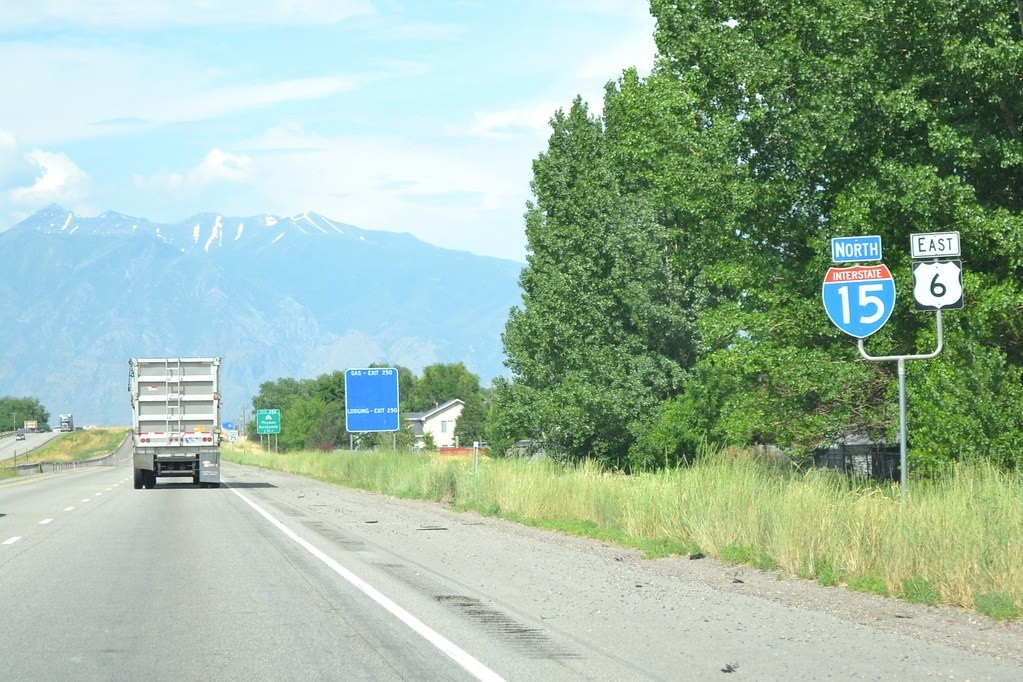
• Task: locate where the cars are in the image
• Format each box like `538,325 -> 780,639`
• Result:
16,435 -> 25,441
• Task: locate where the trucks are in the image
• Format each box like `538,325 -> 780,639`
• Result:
127,357 -> 223,489
59,414 -> 73,432
